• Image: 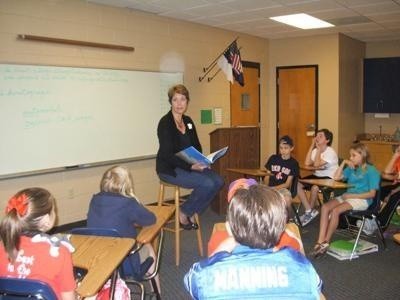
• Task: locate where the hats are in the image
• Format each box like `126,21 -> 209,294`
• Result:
280,135 -> 294,146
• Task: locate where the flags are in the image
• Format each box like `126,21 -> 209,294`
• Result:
218,41 -> 244,88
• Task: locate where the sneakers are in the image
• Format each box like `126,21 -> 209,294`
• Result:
308,239 -> 330,258
357,218 -> 378,236
315,248 -> 328,260
302,207 -> 320,227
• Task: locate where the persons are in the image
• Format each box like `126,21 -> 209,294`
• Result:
264,135 -> 299,191
156,84 -> 225,230
182,185 -> 326,300
295,128 -> 339,227
86,165 -> 163,300
0,187 -> 82,300
356,145 -> 400,236
207,177 -> 303,258
307,142 -> 381,259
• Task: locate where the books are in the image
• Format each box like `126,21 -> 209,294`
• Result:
175,146 -> 228,170
326,239 -> 363,261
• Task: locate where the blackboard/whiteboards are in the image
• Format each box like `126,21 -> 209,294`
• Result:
0,61 -> 185,182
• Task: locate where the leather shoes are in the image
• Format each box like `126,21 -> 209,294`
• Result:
179,220 -> 193,231
188,215 -> 199,230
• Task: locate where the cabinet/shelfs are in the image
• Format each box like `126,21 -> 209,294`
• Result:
361,143 -> 392,170
363,57 -> 400,113
